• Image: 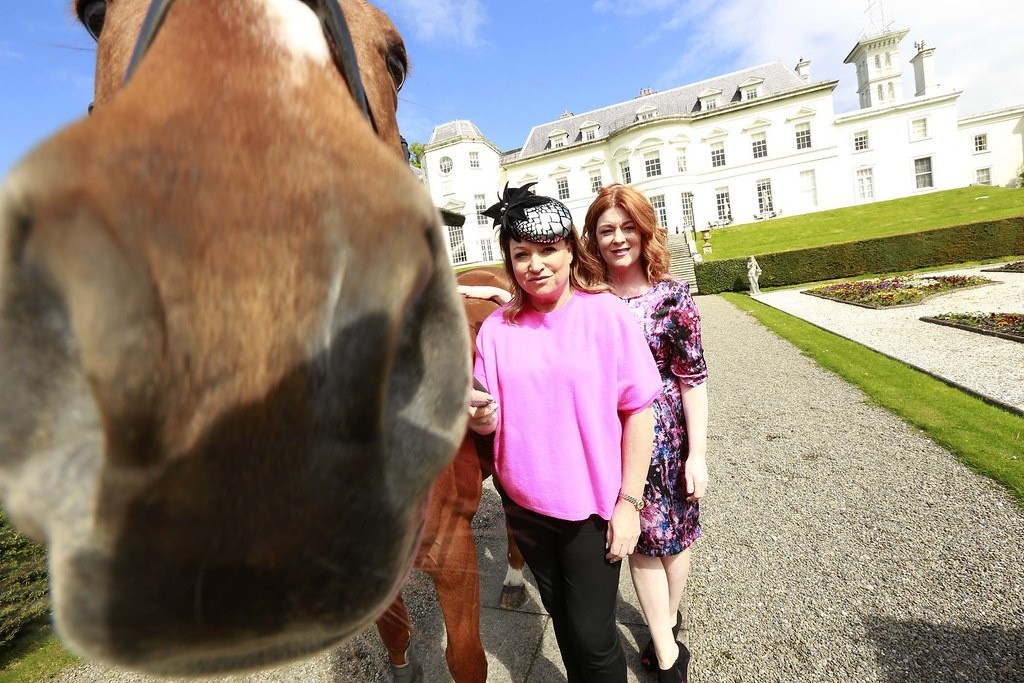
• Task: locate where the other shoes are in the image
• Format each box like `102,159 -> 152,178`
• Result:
655,642 -> 690,683
642,610 -> 684,668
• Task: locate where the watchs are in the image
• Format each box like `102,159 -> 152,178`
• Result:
618,492 -> 645,511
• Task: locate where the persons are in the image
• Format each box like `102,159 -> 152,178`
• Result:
579,182 -> 709,683
467,180 -> 662,683
747,255 -> 762,294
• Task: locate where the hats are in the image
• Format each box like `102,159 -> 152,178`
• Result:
480,182 -> 572,244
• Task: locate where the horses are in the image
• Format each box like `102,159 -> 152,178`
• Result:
0,0 -> 535,683
